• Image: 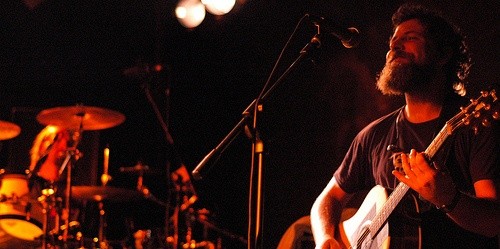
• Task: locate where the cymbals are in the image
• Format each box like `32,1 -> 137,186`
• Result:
120,164 -> 149,172
36,106 -> 125,131
0,119 -> 21,141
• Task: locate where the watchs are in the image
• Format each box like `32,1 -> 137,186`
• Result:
436,187 -> 461,214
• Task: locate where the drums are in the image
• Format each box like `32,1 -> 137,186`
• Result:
0,173 -> 43,241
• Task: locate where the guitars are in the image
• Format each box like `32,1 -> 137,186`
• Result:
338,89 -> 500,249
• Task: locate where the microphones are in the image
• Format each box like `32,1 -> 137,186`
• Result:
306,14 -> 362,49
119,165 -> 150,173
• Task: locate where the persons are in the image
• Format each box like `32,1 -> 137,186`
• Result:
310,3 -> 500,249
27,125 -> 83,201
